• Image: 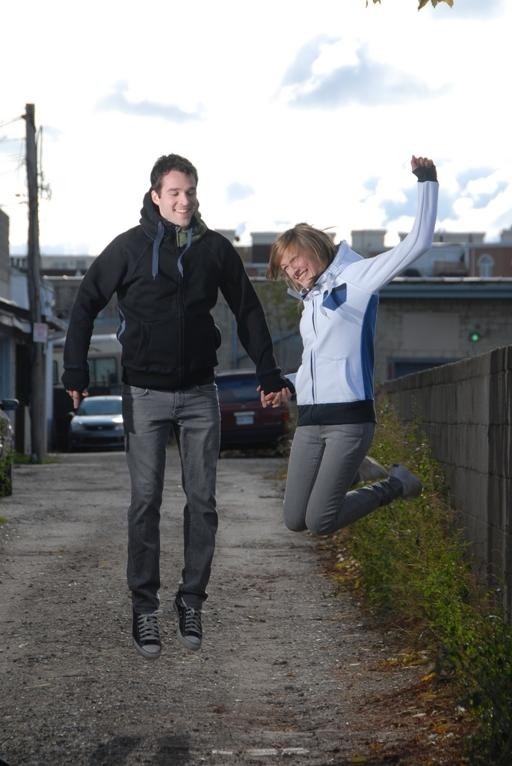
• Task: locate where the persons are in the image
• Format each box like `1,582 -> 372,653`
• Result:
257,154 -> 440,538
62,155 -> 292,661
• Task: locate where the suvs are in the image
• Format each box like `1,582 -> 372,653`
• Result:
216,368 -> 288,452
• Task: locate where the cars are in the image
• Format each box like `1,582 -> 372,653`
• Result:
68,392 -> 126,449
0,397 -> 22,496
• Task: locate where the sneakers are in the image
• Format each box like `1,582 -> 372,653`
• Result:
359,456 -> 388,482
173,594 -> 202,649
132,613 -> 161,658
390,464 -> 423,499
